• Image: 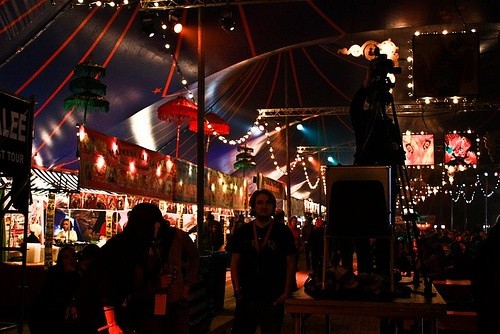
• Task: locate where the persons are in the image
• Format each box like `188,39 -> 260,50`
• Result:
423,139 -> 431,149
231,214 -> 244,236
230,188 -> 296,334
273,208 -> 299,292
57,219 -> 77,241
133,223 -> 200,334
187,214 -> 223,251
307,219 -> 324,270
288,216 -> 301,266
77,245 -> 100,274
348,67 -> 400,280
302,217 -> 314,268
28,246 -> 78,334
76,202 -> 162,334
405,143 -> 413,153
112,212 -> 122,236
470,218 -> 500,334
396,224 -> 470,281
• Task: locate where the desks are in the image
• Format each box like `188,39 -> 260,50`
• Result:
285,276 -> 446,334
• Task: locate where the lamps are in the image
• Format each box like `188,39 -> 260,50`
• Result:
181,76 -> 187,85
51,0 -> 56,7
139,10 -> 155,38
218,0 -> 237,32
168,9 -> 183,33
160,16 -> 168,30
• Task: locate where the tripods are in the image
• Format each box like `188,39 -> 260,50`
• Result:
353,96 -> 431,290
